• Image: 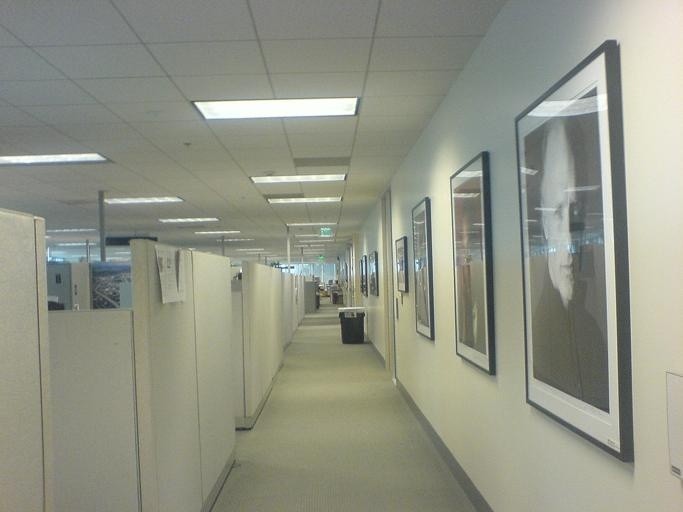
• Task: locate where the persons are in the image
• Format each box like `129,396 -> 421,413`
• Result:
533,113 -> 609,413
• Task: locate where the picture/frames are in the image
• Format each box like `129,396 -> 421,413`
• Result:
395,236 -> 407,293
513,40 -> 633,464
448,151 -> 495,377
411,198 -> 435,341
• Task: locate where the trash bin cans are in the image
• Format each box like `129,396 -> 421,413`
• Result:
332,290 -> 343,304
338,307 -> 365,343
